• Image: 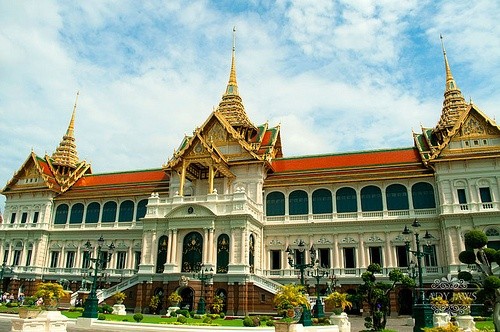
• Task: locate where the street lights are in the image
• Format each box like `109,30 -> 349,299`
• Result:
329,275 -> 341,292
403,217 -> 434,328
286,239 -> 318,326
0,263 -> 13,302
81,235 -> 115,318
307,259 -> 329,319
407,260 -> 419,319
193,263 -> 214,315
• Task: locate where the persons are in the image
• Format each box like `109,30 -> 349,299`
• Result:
3,292 -> 44,306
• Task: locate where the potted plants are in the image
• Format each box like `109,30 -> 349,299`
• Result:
210,295 -> 224,314
431,296 -> 450,313
113,290 -> 126,304
168,291 -> 183,307
19,297 -> 39,318
451,292 -> 474,315
322,291 -> 351,315
273,283 -> 310,323
32,281 -> 67,310
